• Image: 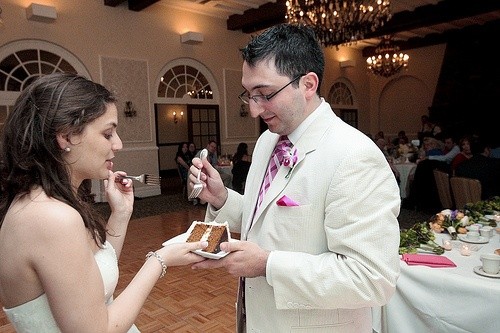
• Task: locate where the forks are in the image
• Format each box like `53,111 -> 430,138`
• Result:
188,149 -> 208,199
115,173 -> 162,186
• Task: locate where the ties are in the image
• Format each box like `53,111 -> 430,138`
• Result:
240,136 -> 292,318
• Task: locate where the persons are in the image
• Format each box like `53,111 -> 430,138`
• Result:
0,73 -> 209,333
232,142 -> 251,168
371,115 -> 500,200
175,140 -> 217,206
188,24 -> 402,333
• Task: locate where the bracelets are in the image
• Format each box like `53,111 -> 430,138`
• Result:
145,250 -> 167,278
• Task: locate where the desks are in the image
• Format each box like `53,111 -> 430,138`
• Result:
370,211 -> 500,333
214,164 -> 233,189
395,162 -> 417,200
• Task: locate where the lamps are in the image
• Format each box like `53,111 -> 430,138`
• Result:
123,100 -> 137,117
26,3 -> 57,24
239,104 -> 248,117
284,0 -> 409,78
173,111 -> 186,127
180,31 -> 204,43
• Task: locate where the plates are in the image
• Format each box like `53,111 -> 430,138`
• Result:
459,234 -> 489,243
474,265 -> 500,278
162,232 -> 239,260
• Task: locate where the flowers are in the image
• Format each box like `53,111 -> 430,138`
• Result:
396,195 -> 500,254
280,141 -> 297,178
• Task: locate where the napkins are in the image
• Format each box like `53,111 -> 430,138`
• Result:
400,254 -> 457,268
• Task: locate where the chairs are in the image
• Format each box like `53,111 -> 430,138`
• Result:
406,159 -> 481,209
173,157 -> 188,199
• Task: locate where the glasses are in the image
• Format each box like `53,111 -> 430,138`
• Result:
238,73 -> 308,106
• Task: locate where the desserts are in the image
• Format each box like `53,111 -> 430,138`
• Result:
185,223 -> 229,254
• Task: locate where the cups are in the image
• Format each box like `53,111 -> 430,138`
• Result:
480,254 -> 500,275
460,243 -> 470,256
465,224 -> 500,241
442,236 -> 452,251
